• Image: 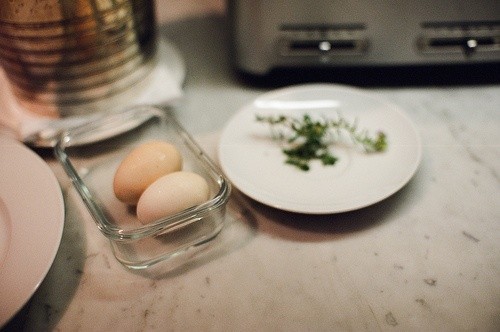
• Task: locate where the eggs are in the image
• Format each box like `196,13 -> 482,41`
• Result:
136,171 -> 210,226
114,140 -> 181,205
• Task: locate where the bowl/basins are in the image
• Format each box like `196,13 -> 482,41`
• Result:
55,104 -> 234,272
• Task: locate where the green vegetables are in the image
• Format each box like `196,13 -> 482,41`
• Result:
255,112 -> 387,172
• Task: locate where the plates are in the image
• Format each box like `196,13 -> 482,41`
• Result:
0,33 -> 190,149
220,83 -> 423,214
0,129 -> 67,325
